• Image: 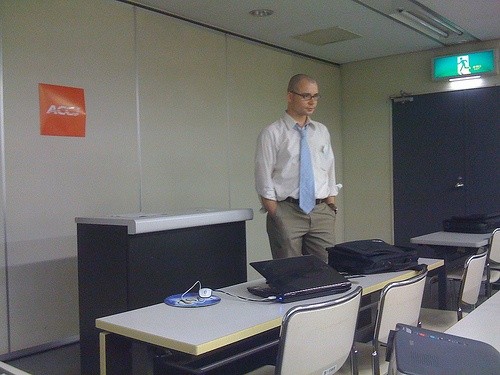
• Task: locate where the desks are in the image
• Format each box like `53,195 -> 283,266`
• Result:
96,257 -> 445,375
409,230 -> 494,301
441,290 -> 500,353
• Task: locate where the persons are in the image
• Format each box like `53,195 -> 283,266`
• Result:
254,73 -> 338,264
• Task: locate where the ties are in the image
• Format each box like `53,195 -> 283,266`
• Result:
298,124 -> 316,214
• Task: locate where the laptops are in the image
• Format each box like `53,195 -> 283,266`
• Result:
246,254 -> 352,302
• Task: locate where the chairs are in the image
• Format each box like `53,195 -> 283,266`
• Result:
242,227 -> 500,375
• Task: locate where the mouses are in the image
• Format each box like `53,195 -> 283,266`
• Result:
199,288 -> 212,297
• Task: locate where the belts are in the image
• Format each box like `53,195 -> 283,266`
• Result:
284,196 -> 328,205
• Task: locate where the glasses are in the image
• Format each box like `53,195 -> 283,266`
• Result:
288,89 -> 322,101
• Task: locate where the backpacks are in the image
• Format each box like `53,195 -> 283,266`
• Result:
325,239 -> 428,274
442,214 -> 500,233
